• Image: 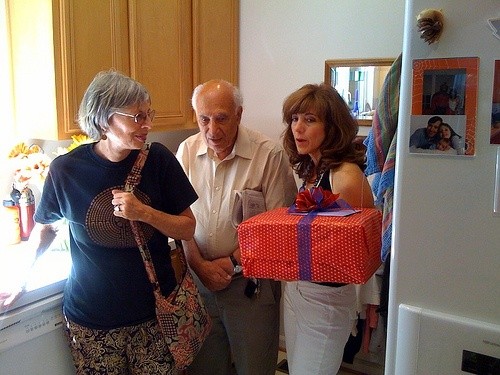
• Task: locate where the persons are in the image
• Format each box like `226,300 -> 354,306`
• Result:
409,116 -> 465,155
0,72 -> 199,375
175,78 -> 298,375
275,84 -> 374,375
430,82 -> 450,114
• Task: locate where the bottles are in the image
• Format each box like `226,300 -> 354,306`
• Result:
1,185 -> 35,244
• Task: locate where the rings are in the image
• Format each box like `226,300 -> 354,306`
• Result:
118,205 -> 120,211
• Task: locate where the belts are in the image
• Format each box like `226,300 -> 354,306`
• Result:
315,281 -> 349,288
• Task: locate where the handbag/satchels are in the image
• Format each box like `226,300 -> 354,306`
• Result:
156,269 -> 213,369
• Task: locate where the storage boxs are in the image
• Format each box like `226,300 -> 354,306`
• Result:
236,184 -> 384,284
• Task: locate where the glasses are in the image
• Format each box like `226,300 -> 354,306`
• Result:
115,110 -> 156,124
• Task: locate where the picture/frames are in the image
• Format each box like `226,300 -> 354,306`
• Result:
407,55 -> 479,156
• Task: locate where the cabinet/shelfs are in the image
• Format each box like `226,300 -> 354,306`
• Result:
4,0 -> 241,141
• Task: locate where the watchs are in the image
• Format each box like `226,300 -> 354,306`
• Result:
230,254 -> 242,273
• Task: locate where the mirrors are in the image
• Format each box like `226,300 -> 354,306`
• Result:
325,57 -> 396,127
